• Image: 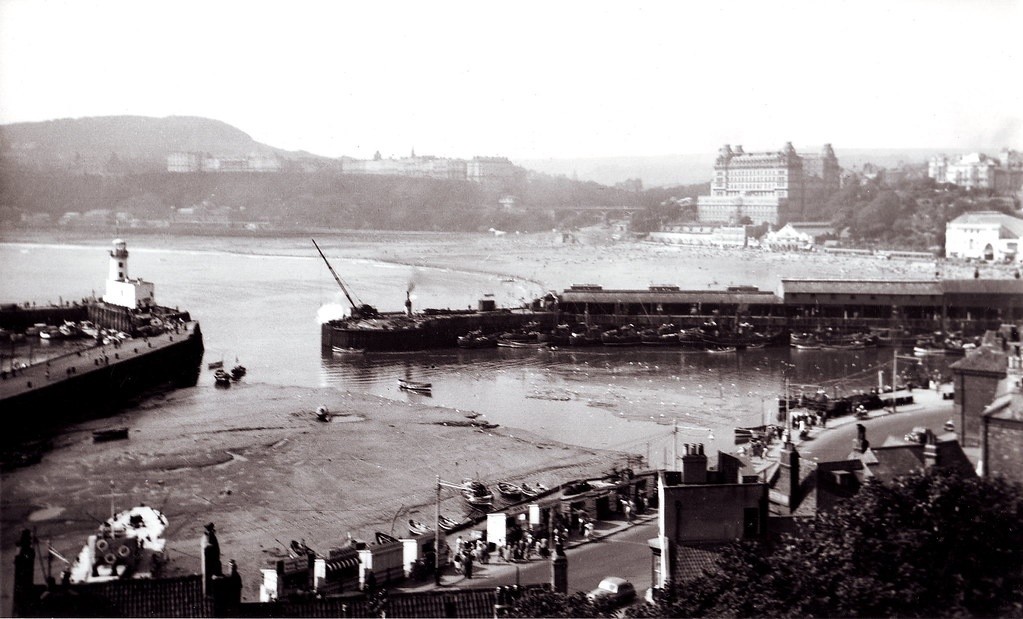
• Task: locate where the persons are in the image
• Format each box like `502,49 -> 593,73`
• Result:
553,494 -> 650,542
455,529 -> 550,564
750,408 -> 827,460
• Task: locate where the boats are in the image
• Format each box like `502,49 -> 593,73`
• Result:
438,514 -> 459,530
460,478 -> 494,507
495,480 -> 522,499
453,295 -> 976,356
287,539 -> 327,565
375,531 -> 399,546
735,423 -> 783,443
520,483 -> 538,497
214,369 -> 230,384
408,518 -> 435,537
26,319 -> 132,345
91,427 -> 129,442
398,378 -> 432,394
